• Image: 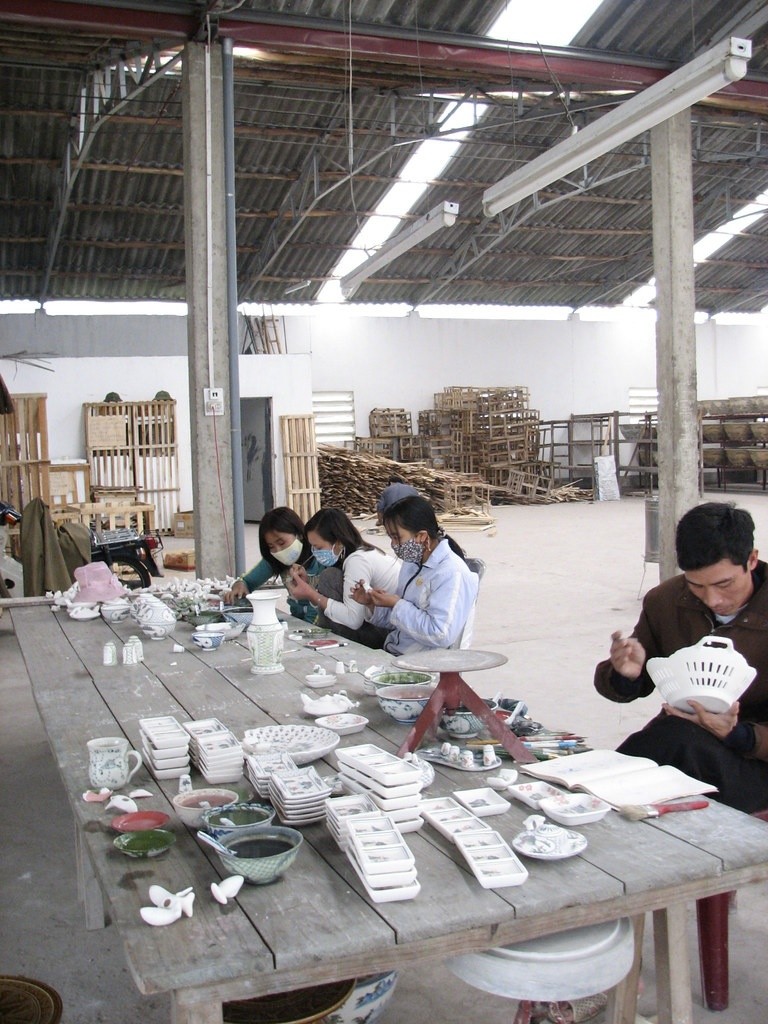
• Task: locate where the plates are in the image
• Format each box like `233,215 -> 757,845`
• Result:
112,829 -> 176,857
137,712 -> 588,903
69,612 -> 100,621
111,811 -> 170,833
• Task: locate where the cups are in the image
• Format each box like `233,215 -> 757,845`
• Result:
86,737 -> 142,791
173,645 -> 185,653
178,774 -> 193,794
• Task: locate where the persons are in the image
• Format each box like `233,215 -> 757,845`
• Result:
282,507 -> 402,650
593,503 -> 768,818
348,495 -> 476,657
223,507 -> 320,625
375,477 -> 418,525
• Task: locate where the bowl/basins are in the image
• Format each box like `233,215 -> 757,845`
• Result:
369,671 -> 435,692
214,826 -> 303,885
374,685 -> 437,722
438,698 -> 499,739
195,622 -> 246,641
200,803 -> 276,841
192,631 -> 225,651
172,788 -> 239,829
101,605 -> 131,623
220,607 -> 254,628
617,396 -> 768,467
538,793 -> 611,827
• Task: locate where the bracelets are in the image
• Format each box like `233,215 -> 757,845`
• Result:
317,595 -> 323,608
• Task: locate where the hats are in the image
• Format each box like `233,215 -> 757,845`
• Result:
71,561 -> 128,602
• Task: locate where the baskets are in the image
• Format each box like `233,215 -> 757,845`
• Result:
646,636 -> 758,714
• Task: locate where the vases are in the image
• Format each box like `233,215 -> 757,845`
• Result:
245,593 -> 285,675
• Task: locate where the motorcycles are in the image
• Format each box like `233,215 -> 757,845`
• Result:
0,501 -> 165,598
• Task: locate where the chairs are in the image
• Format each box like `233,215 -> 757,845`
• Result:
449,556 -> 487,650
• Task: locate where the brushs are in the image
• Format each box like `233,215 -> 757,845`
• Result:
620,801 -> 709,822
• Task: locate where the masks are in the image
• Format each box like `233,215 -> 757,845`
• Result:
311,544 -> 345,566
270,534 -> 303,566
391,530 -> 429,563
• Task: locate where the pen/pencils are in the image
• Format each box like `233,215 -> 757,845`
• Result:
314,643 -> 350,651
293,629 -> 333,633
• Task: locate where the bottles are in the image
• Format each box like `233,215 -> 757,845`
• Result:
246,592 -> 285,675
103,635 -> 144,666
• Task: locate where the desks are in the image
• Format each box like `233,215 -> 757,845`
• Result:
10,596 -> 768,1024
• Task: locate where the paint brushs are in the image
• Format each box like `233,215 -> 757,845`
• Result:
298,555 -> 313,570
353,580 -> 363,588
465,732 -> 588,760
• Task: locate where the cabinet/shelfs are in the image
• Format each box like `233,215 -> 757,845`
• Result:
539,407 -> 768,496
83,400 -> 180,536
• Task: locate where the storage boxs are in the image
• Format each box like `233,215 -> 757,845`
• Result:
163,547 -> 197,572
174,510 -> 194,539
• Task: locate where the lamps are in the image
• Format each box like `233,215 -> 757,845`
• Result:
284,279 -> 312,296
482,0 -> 752,218
339,0 -> 458,290
